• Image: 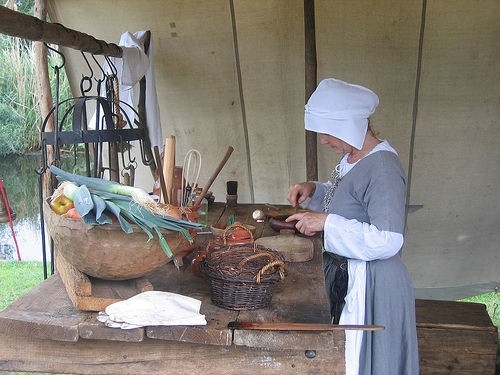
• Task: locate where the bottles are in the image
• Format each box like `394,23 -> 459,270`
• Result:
188,188 -> 208,229
191,181 -> 255,278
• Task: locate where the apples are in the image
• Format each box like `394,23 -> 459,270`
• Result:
50,195 -> 81,220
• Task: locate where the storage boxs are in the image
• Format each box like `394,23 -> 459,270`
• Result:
414,299 -> 498,375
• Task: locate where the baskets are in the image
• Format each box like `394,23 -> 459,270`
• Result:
199,225 -> 289,312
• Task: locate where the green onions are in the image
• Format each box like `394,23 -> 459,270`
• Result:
50,165 -> 206,258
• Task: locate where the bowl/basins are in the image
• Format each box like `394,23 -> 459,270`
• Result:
209,223 -> 256,237
268,213 -> 300,233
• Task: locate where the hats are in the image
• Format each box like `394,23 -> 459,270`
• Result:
303,77 -> 380,150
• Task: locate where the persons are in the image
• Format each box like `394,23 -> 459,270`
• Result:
287,78 -> 420,375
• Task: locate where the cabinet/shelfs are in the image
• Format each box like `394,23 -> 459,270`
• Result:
0,202 -> 346,375
